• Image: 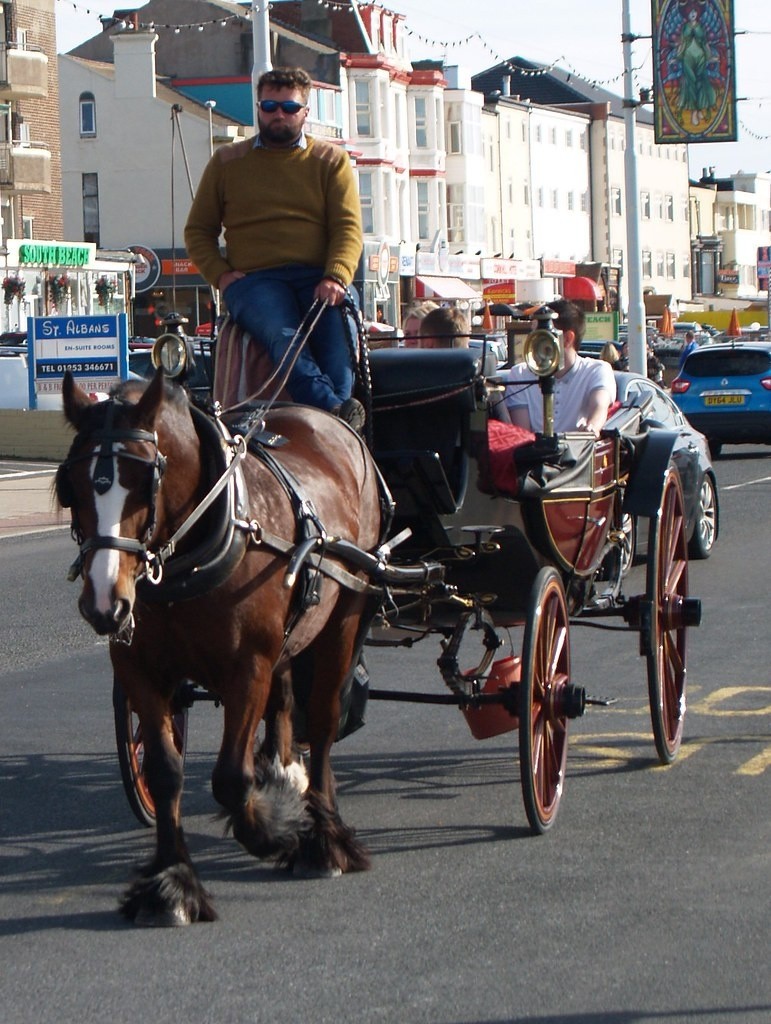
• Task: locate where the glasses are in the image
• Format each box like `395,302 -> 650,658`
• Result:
258,100 -> 305,114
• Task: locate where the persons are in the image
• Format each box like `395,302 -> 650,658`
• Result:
600,342 -> 629,370
404,300 -> 440,349
647,343 -> 665,389
619,342 -> 629,366
184,65 -> 366,446
504,298 -> 617,438
420,306 -> 469,348
679,331 -> 700,370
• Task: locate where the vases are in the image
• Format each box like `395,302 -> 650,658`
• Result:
4,290 -> 15,304
99,293 -> 109,306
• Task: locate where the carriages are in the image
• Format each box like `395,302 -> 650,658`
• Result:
33,333 -> 702,930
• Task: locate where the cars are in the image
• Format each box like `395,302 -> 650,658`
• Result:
616,371 -> 718,560
366,320 -> 770,363
672,341 -> 771,461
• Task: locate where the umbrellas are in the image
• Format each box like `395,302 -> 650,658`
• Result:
725,307 -> 742,338
476,303 -> 525,328
511,303 -> 536,321
659,305 -> 675,337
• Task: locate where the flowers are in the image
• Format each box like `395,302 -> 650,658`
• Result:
49,275 -> 71,311
1,277 -> 25,302
95,278 -> 117,293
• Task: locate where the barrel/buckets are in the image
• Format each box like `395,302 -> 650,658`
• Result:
459,626 -> 522,738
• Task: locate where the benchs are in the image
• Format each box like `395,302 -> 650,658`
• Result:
200,340 -> 475,514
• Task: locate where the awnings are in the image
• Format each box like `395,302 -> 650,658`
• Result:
416,277 -> 482,300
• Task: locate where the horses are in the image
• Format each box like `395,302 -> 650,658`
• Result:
50,364 -> 382,929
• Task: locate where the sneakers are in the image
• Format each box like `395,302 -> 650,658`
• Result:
333,398 -> 366,444
